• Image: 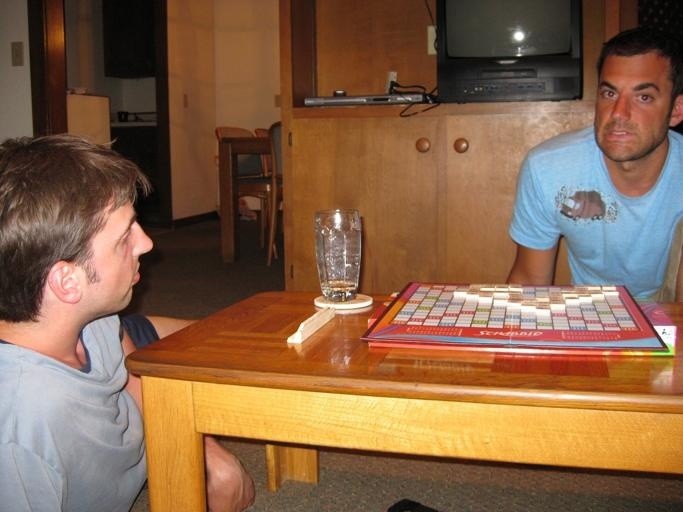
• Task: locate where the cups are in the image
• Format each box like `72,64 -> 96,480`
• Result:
313,207 -> 363,302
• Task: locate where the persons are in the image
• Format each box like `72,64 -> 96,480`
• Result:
0,134 -> 256,512
505,28 -> 682,308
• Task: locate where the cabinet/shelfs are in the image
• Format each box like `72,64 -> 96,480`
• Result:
278,0 -> 621,289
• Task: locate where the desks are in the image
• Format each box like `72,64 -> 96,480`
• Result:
124,291 -> 683,512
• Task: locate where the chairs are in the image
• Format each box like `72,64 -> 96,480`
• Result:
213,122 -> 281,265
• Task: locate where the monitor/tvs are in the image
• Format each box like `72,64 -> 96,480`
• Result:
435,0 -> 586,105
